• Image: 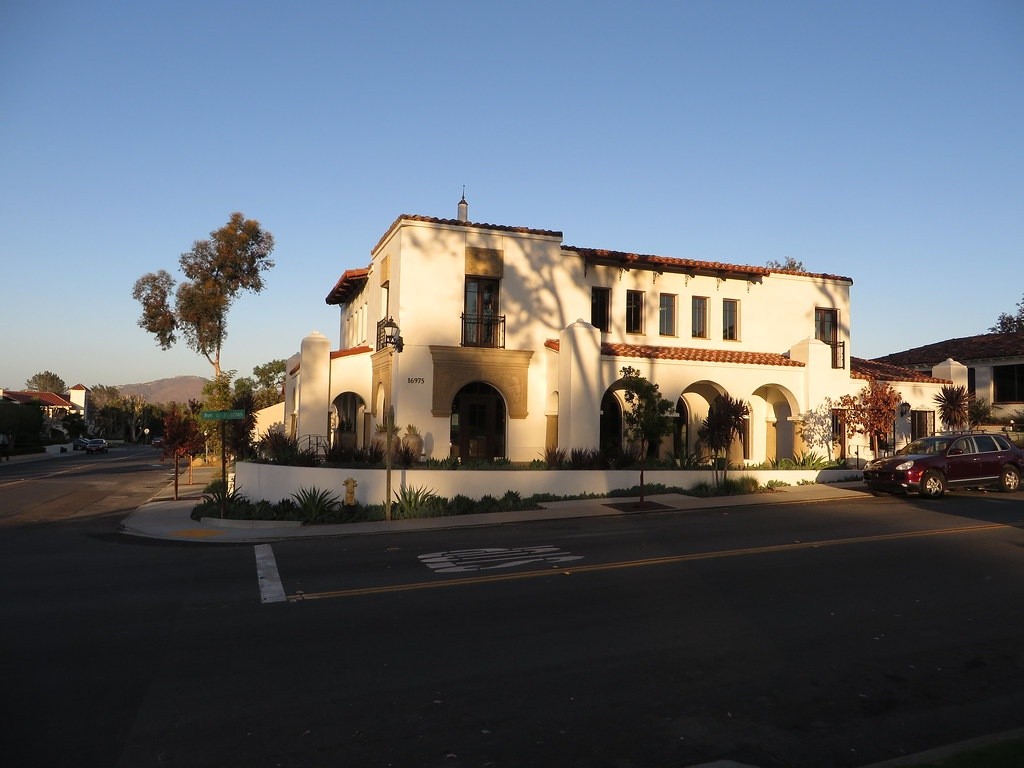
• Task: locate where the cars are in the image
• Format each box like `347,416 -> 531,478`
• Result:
86,439 -> 109,454
72,439 -> 88,450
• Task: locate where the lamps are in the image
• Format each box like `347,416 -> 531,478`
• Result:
381,316 -> 405,353
900,400 -> 911,417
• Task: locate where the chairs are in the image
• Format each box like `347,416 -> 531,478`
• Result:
938,442 -> 947,451
953,440 -> 969,454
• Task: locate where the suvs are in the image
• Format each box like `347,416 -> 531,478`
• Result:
863,429 -> 1023,497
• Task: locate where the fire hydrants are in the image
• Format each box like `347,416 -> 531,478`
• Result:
343,478 -> 358,504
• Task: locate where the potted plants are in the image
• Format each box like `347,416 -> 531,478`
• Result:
334,416 -> 357,455
630,429 -> 644,458
694,427 -> 713,463
371,424 -> 401,463
832,433 -> 843,461
402,424 -> 424,463
